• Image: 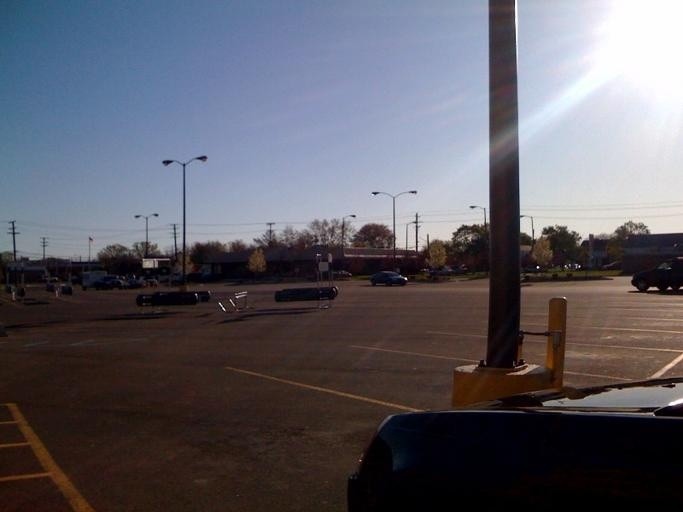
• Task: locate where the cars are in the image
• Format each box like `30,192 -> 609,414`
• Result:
307,270 -> 352,280
431,264 -> 468,276
630,255 -> 682,295
601,261 -> 623,271
80,271 -> 185,291
369,270 -> 408,287
346,373 -> 683,512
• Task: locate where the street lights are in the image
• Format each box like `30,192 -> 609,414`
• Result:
161,156 -> 205,286
87,237 -> 94,269
341,215 -> 356,268
405,221 -> 417,252
266,222 -> 275,242
134,212 -> 159,259
519,215 -> 535,248
469,205 -> 486,233
371,190 -> 416,271
